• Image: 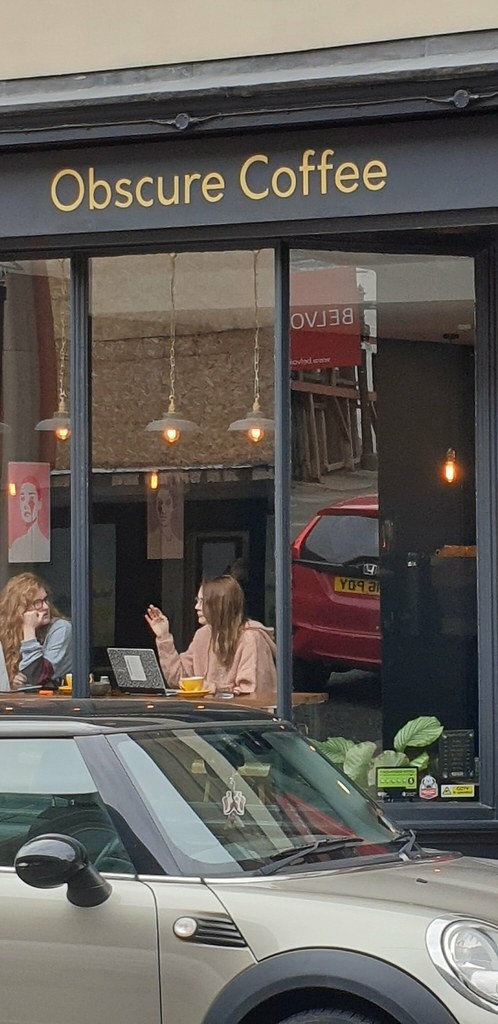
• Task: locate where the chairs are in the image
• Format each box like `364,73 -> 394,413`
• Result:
13,746 -> 136,874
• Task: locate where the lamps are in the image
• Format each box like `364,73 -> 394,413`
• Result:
34,249 -> 276,442
443,332 -> 460,484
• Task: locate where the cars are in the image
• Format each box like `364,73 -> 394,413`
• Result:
290,491 -> 381,692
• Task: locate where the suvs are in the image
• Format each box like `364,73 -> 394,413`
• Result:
0,688 -> 498,1024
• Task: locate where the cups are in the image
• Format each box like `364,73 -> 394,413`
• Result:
181,676 -> 205,691
66,674 -> 93,688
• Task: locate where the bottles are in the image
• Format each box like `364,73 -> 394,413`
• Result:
101,676 -> 111,693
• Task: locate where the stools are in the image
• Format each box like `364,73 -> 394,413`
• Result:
192,758 -> 272,805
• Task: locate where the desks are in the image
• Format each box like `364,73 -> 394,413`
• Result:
0,686 -> 329,744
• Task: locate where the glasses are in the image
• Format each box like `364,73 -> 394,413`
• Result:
27,594 -> 51,610
195,596 -> 203,606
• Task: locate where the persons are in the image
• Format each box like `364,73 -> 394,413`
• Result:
0,573 -> 73,692
145,573 -> 279,697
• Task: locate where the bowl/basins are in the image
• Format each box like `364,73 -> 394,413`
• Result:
90,682 -> 110,696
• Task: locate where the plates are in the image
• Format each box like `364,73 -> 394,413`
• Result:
176,690 -> 211,698
58,686 -> 72,694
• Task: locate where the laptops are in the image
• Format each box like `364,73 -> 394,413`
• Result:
0,642 -> 43,694
107,647 -> 181,697
